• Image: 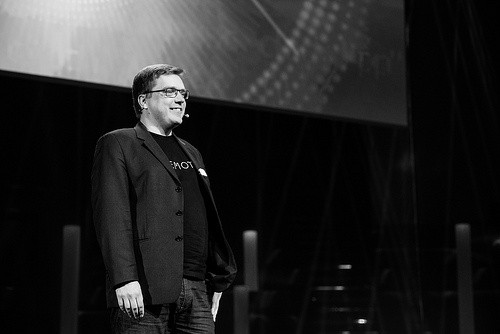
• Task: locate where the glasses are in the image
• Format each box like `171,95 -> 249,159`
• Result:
143,88 -> 190,99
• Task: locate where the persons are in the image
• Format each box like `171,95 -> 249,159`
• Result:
92,63 -> 237,334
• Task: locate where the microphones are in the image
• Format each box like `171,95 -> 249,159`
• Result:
184,113 -> 190,118
